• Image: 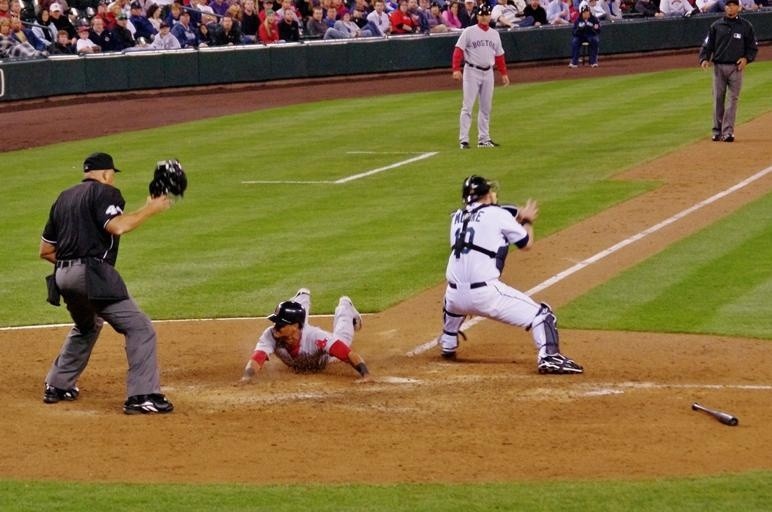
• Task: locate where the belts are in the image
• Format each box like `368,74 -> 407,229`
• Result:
56,258 -> 88,267
466,61 -> 490,71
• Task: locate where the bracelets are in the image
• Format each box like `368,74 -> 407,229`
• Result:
521,218 -> 531,226
355,362 -> 369,377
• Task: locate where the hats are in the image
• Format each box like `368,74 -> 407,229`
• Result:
84,153 -> 122,173
8,5 -> 193,35
50,3 -> 61,12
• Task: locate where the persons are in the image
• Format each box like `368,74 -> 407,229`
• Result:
491,1 -> 765,29
39,150 -> 174,415
451,4 -> 510,148
0,0 -> 475,62
439,174 -> 584,374
567,6 -> 600,69
241,288 -> 377,383
698,0 -> 759,142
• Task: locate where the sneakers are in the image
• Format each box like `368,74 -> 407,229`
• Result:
537,356 -> 584,373
712,134 -> 735,142
339,296 -> 363,330
43,384 -> 79,402
124,393 -> 173,414
478,140 -> 499,148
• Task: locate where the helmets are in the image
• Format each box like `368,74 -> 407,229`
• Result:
462,175 -> 491,203
478,3 -> 492,15
267,301 -> 305,327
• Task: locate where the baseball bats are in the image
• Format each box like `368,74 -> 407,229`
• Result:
692,403 -> 738,425
